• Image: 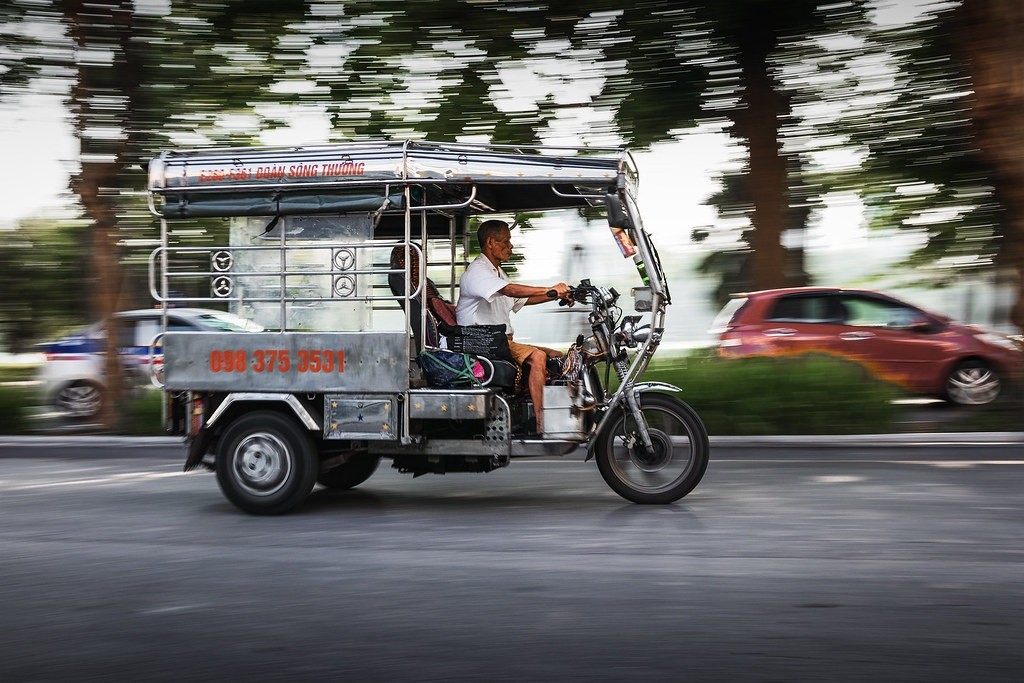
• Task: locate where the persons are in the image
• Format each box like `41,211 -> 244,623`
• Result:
455,219 -> 575,436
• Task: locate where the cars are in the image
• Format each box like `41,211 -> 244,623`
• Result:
705,285 -> 1024,408
40,306 -> 275,423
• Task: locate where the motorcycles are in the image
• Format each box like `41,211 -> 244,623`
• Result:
144,135 -> 712,519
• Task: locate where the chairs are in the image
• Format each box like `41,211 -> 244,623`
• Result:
388,240 -> 517,391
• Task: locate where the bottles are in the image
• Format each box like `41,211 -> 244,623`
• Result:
632,254 -> 650,286
609,227 -> 636,258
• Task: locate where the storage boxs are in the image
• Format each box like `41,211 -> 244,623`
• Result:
409,388 -> 489,420
